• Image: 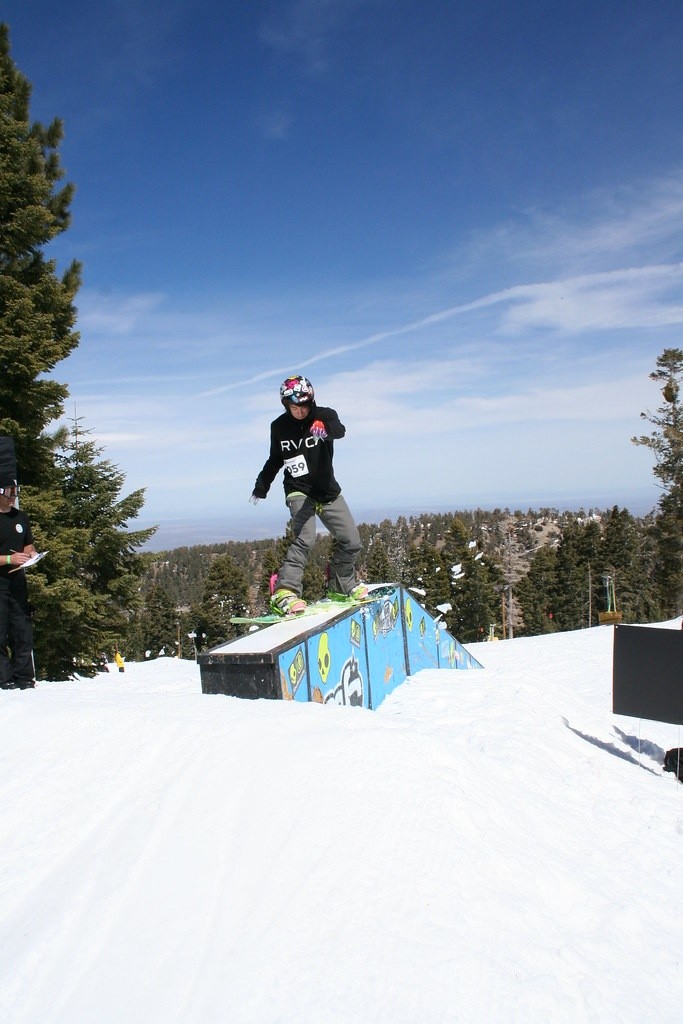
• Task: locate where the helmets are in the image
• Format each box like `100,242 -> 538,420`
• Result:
280,375 -> 314,405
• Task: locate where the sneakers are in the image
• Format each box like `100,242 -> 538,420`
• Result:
339,587 -> 369,600
270,590 -> 305,613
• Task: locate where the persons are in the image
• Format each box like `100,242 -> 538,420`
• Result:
0,474 -> 39,689
249,376 -> 369,614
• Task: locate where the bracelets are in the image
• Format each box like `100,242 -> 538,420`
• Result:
7,555 -> 11,564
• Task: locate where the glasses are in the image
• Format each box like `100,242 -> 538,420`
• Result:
0,487 -> 20,498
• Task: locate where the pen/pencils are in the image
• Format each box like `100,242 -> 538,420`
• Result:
10,549 -> 16,552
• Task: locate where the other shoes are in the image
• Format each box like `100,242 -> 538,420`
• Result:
0,681 -> 16,689
18,679 -> 35,690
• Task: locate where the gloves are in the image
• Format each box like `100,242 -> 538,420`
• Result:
250,496 -> 260,505
309,421 -> 329,442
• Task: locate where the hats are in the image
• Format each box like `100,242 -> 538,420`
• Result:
0,475 -> 18,488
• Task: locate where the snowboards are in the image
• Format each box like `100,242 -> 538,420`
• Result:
229,588 -> 388,626
